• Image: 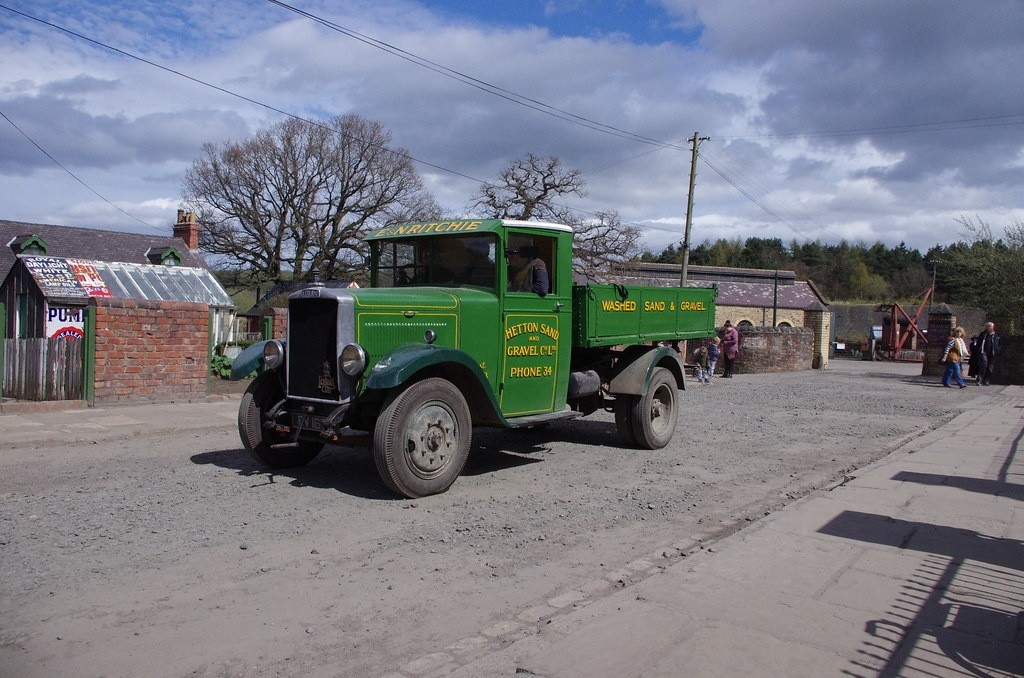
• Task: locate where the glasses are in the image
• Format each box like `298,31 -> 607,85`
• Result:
956,331 -> 961,333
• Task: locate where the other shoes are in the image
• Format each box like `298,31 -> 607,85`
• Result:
976,381 -> 981,386
705,378 -> 710,383
983,381 -> 990,386
719,373 -> 733,378
709,374 -> 717,379
698,379 -> 702,383
960,385 -> 966,389
943,384 -> 952,388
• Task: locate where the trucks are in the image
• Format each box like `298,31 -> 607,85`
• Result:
230,219 -> 716,494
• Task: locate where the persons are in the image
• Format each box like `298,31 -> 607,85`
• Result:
505,234 -> 549,296
941,326 -> 971,389
968,335 -> 979,378
709,336 -> 721,378
722,320 -> 738,378
409,240 -> 496,288
976,322 -> 1000,386
693,346 -> 710,383
